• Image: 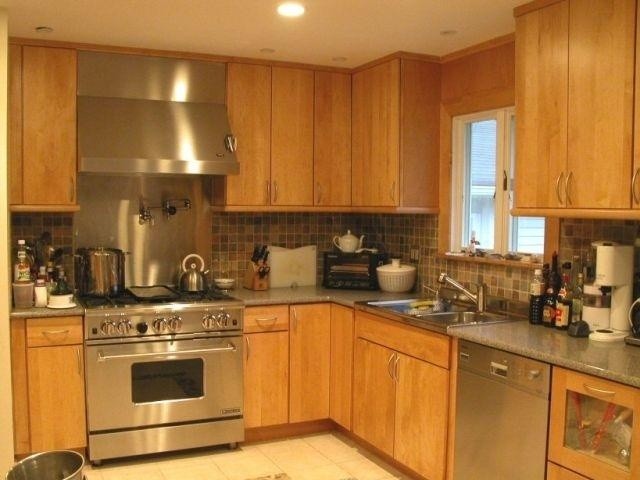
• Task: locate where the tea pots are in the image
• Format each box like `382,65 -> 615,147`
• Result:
333,230 -> 365,254
177,254 -> 212,291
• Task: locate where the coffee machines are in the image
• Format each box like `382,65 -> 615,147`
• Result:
589,244 -> 633,344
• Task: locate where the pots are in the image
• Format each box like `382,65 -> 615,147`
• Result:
71,242 -> 131,299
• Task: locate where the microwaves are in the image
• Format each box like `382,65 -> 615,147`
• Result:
324,249 -> 387,292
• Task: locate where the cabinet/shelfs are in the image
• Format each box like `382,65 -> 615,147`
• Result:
510,0 -> 640,220
544,365 -> 640,480
352,50 -> 439,216
352,310 -> 458,479
211,61 -> 314,214
315,63 -> 353,212
244,305 -> 289,445
331,302 -> 352,442
9,316 -> 87,463
290,302 -> 331,438
8,37 -> 81,213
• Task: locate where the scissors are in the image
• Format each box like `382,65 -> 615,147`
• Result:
258,266 -> 270,279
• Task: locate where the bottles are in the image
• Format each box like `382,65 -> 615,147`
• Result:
529,252 -> 594,332
11,240 -> 75,309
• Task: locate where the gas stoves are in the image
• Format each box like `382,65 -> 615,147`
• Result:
72,284 -> 241,341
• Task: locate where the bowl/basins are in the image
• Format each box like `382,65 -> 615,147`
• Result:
215,277 -> 236,290
376,267 -> 416,292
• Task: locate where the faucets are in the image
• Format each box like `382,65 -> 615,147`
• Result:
436,272 -> 488,312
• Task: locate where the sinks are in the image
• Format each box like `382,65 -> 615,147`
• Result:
366,298 -> 473,316
409,311 -> 511,329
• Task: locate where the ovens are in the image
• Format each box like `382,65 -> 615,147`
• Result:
85,331 -> 246,467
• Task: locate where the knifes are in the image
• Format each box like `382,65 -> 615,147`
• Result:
252,242 -> 269,264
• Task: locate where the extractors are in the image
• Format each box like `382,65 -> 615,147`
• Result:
75,45 -> 242,181
